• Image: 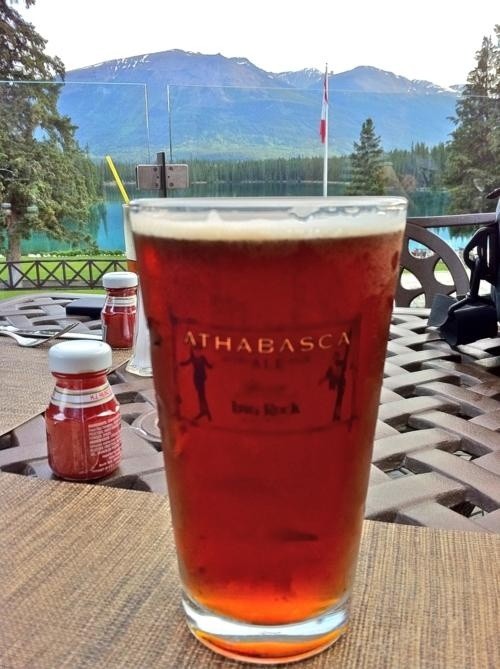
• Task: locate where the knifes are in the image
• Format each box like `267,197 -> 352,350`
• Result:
0,326 -> 104,340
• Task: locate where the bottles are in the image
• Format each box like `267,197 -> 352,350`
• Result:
45,339 -> 123,483
98,270 -> 137,350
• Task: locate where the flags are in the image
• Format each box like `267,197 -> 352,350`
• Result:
320,69 -> 329,144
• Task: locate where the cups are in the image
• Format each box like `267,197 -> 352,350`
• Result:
116,197 -> 412,667
118,198 -> 145,277
124,292 -> 153,377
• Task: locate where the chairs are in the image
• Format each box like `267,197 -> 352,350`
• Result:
393,221 -> 469,307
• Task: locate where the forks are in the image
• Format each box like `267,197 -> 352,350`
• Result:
0,320 -> 78,348
